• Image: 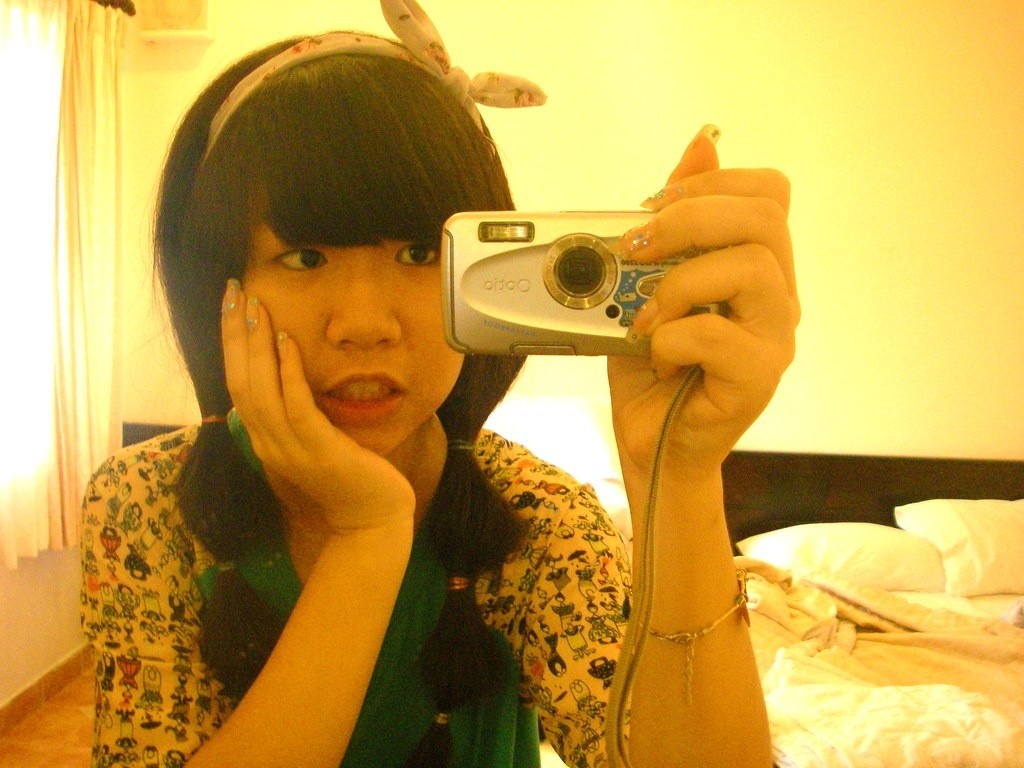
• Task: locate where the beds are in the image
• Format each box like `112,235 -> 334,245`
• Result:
721,451 -> 1024,768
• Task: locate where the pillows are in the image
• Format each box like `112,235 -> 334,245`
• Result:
735,522 -> 945,595
894,499 -> 1024,597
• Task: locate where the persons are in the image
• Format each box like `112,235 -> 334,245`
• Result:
81,0 -> 800,768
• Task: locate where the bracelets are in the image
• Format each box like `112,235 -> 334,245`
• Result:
630,569 -> 750,710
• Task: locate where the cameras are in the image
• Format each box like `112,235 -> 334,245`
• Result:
440,208 -> 722,356
